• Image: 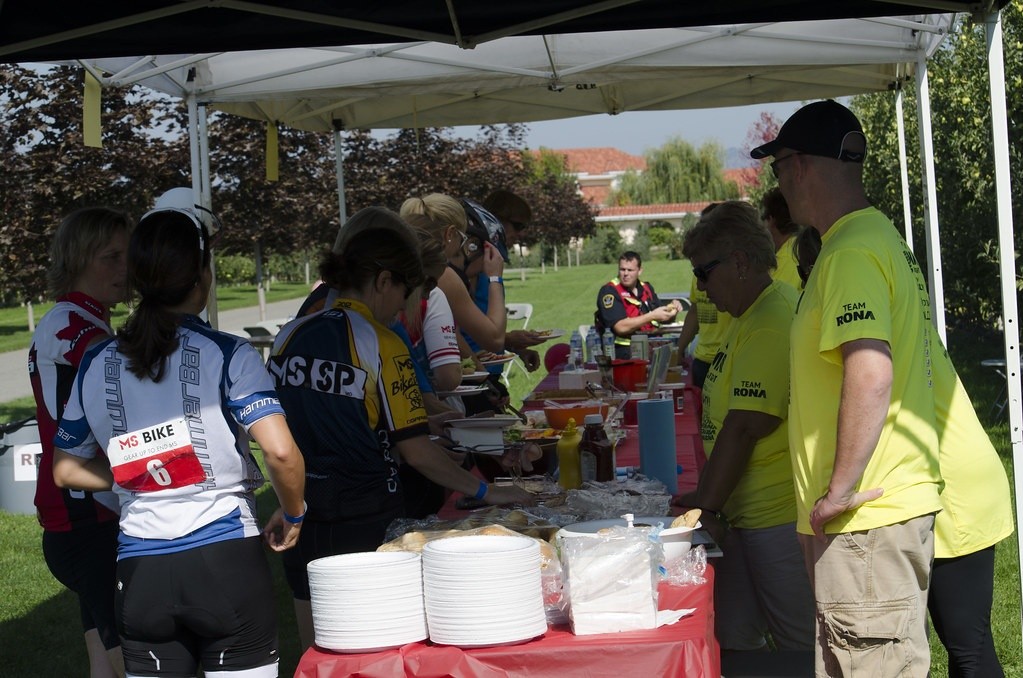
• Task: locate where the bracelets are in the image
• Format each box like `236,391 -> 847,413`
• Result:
283,498 -> 308,522
474,480 -> 488,500
488,276 -> 505,284
693,505 -> 721,520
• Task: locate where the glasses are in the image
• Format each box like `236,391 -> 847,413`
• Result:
453,228 -> 471,249
375,258 -> 414,301
139,207 -> 205,268
426,275 -> 438,292
194,204 -> 225,248
495,213 -> 531,234
693,256 -> 730,282
770,152 -> 816,178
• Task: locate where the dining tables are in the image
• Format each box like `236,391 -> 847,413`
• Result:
294,361 -> 722,678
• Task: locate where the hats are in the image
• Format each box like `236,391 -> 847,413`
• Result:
750,98 -> 867,164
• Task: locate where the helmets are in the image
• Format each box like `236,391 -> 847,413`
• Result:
456,197 -> 510,263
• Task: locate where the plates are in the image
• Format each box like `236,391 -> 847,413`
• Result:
307,535 -> 548,654
462,371 -> 490,384
529,329 -> 567,338
436,385 -> 489,396
443,417 -> 523,429
481,356 -> 519,366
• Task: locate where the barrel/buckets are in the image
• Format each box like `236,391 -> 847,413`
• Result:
613,359 -> 650,392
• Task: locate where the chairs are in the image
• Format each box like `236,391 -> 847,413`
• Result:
659,298 -> 693,311
500,304 -> 533,386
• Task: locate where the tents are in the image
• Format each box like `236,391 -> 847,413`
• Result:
1,0 -> 1023,460
49,11 -> 963,354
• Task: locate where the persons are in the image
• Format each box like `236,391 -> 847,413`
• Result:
26,189 -> 308,678
260,190 -> 547,654
666,190 -> 817,678
750,95 -> 943,678
789,228 -> 1015,678
595,252 -> 684,357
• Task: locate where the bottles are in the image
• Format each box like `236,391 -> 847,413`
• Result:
556,417 -> 582,490
603,328 -> 615,361
589,326 -> 603,356
620,393 -> 648,427
629,335 -> 648,359
578,413 -> 615,482
570,330 -> 584,371
586,330 -> 598,365
658,383 -> 686,415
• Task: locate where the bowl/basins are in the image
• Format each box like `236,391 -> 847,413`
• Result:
558,516 -> 702,564
543,403 -> 611,431
